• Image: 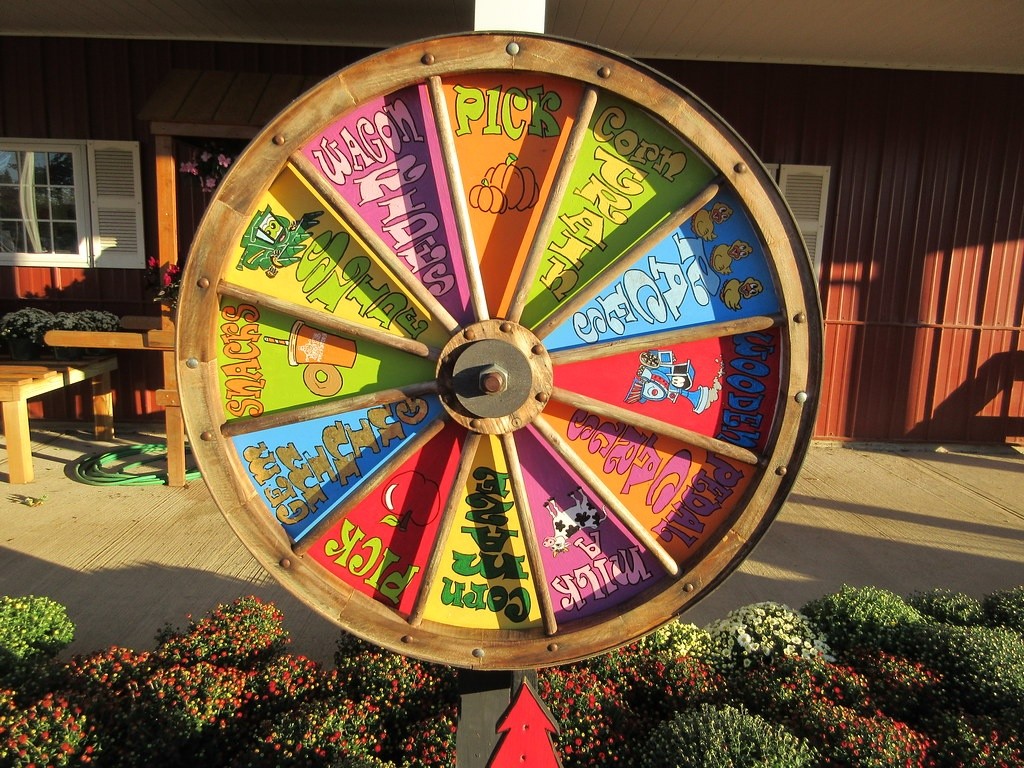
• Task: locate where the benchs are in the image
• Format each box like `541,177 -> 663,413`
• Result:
1,355 -> 119,484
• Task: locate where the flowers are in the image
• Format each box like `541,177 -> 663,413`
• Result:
138,255 -> 182,312
178,138 -> 233,194
